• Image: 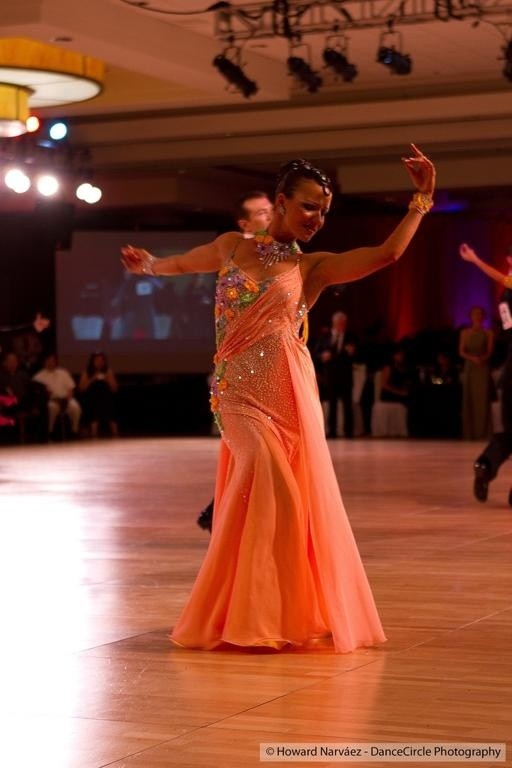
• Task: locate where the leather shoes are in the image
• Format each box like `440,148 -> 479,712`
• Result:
471,462 -> 492,502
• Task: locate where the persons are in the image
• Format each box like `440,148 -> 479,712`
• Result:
77,349 -> 123,440
315,310 -> 358,438
115,140 -> 436,653
193,191 -> 275,536
376,239 -> 512,506
0,350 -> 47,446
31,353 -> 85,441
1,312 -> 52,340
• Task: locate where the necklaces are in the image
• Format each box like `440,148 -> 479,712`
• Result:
253,229 -> 299,271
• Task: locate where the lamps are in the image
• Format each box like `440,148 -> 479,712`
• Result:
0,38 -> 101,142
205,1 -> 414,100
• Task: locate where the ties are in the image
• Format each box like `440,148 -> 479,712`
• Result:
333,334 -> 340,352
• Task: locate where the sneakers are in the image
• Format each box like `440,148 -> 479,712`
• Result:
198,506 -> 213,533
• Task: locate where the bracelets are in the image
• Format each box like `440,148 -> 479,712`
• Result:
407,191 -> 435,219
141,253 -> 157,280
